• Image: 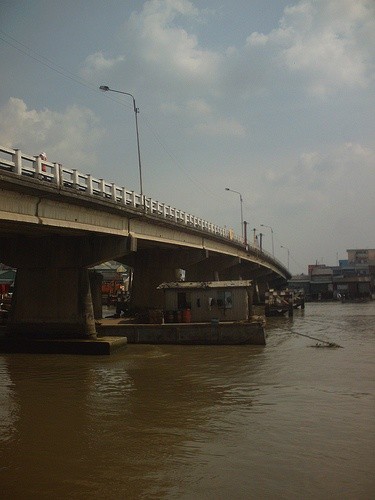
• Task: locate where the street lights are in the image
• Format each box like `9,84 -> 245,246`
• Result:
100,85 -> 143,195
280,245 -> 290,272
225,187 -> 244,239
260,224 -> 274,257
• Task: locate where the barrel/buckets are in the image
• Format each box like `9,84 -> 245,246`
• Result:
167,311 -> 176,321
182,308 -> 190,322
175,311 -> 183,321
